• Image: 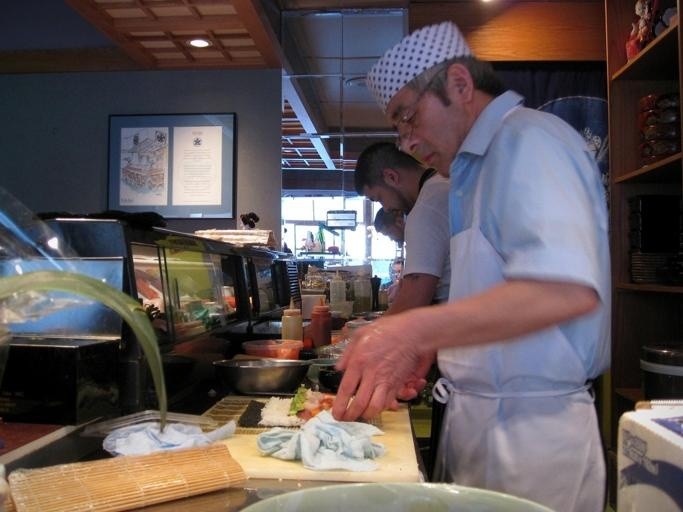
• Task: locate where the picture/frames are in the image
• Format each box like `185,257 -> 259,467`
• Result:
105,104 -> 238,223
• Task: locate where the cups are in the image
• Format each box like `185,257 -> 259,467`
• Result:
371,275 -> 381,311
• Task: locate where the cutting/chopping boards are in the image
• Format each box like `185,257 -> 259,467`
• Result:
199,394 -> 425,486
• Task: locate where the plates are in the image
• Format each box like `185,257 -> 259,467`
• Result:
629,250 -> 671,282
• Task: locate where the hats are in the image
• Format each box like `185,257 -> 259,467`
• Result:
365,23 -> 471,113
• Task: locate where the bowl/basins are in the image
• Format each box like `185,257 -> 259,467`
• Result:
210,356 -> 311,394
242,338 -> 303,360
304,357 -> 340,385
330,301 -> 353,317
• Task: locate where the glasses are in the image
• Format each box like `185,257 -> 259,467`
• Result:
396,68 -> 446,150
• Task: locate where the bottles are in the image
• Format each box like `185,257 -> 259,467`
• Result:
331,311 -> 346,331
280,297 -> 303,340
329,270 -> 347,306
352,270 -> 371,312
377,285 -> 388,310
310,297 -> 332,347
316,227 -> 326,253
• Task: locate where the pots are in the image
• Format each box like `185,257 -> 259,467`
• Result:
639,339 -> 682,403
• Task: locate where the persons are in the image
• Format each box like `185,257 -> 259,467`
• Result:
332,18 -> 611,512
353,139 -> 451,473
374,207 -> 408,248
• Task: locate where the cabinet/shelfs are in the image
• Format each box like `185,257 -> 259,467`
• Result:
601,0 -> 683,462
